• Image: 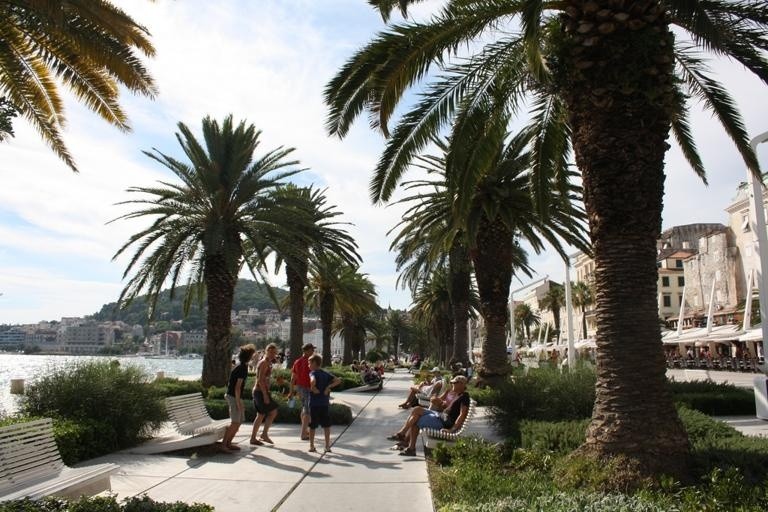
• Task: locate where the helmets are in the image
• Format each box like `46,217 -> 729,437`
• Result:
301,343 -> 317,352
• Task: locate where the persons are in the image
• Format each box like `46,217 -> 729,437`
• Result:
219,343 -> 256,455
668,343 -> 764,363
451,361 -> 467,378
399,366 -> 443,409
387,375 -> 470,457
351,358 -> 395,388
385,372 -> 463,451
249,342 -> 280,447
287,342 -> 317,440
307,353 -> 342,452
506,346 -> 597,365
232,345 -> 291,372
405,353 -> 419,373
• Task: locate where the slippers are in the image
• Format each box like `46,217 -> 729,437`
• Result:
250,437 -> 273,445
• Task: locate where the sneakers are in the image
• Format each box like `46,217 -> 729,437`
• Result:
220,444 -> 240,454
302,432 -> 331,452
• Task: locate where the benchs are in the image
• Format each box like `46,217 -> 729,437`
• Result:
0,418 -> 121,500
162,392 -> 231,451
416,376 -> 478,450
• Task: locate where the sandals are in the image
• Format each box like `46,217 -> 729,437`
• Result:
387,433 -> 416,456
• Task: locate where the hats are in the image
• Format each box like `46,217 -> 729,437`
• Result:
429,367 -> 440,373
450,375 -> 467,383
